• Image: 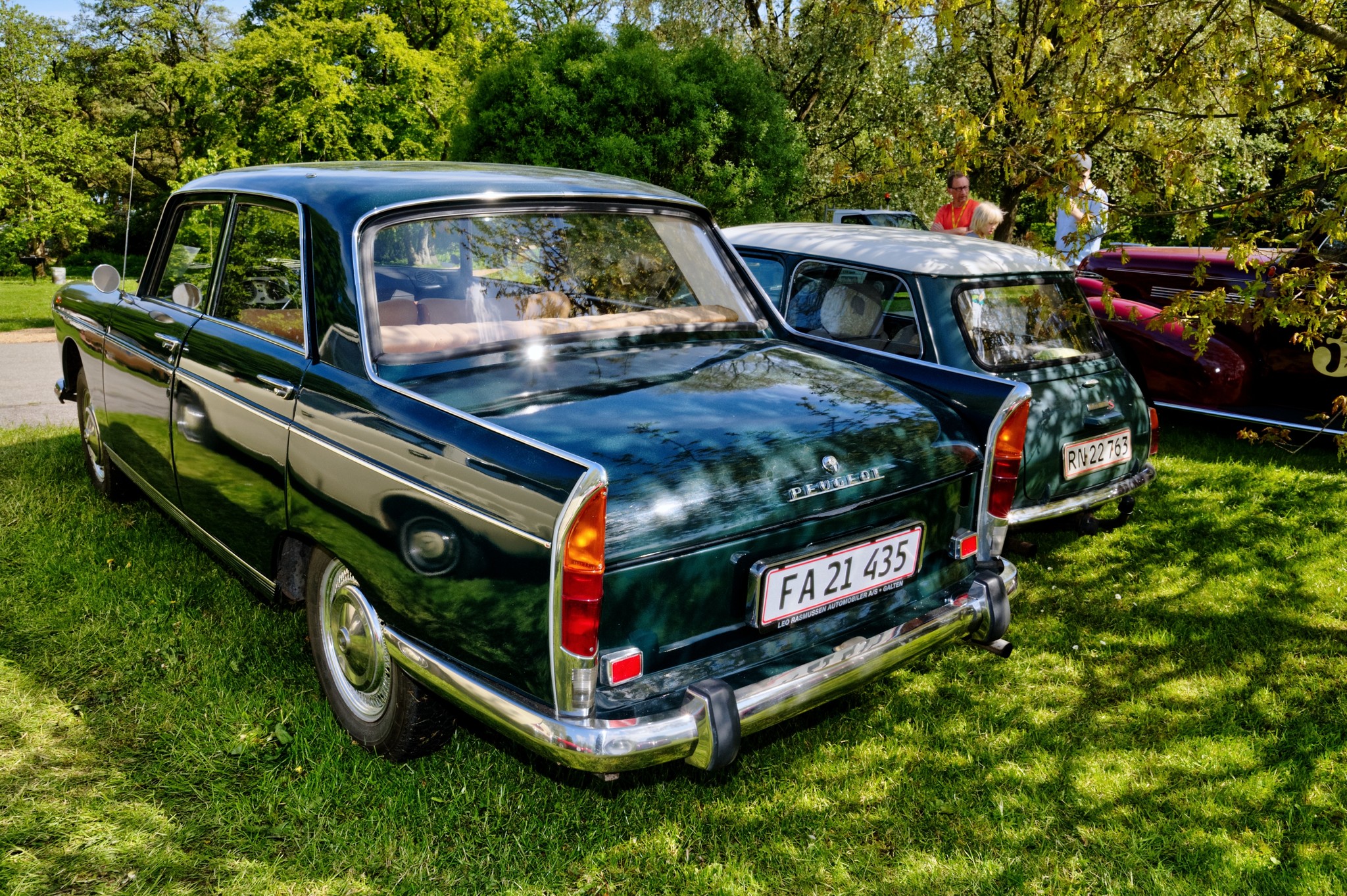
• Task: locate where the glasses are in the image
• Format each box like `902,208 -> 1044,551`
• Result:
950,185 -> 969,192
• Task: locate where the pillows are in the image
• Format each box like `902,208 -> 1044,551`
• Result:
1033,347 -> 1085,360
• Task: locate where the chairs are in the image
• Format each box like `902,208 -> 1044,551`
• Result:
239,298 -> 418,345
806,284 -> 889,352
888,290 -> 985,365
421,292 -> 571,327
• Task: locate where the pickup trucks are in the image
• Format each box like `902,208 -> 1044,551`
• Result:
822,203 -> 930,233
49,159 -> 1035,783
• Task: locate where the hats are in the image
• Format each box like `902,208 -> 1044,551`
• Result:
1068,153 -> 1092,170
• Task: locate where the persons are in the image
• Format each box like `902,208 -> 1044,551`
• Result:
968,201 -> 1002,240
931,173 -> 982,235
1053,153 -> 1108,269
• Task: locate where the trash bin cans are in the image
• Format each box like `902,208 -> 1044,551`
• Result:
51,267 -> 66,284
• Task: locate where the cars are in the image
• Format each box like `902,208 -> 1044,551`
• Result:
1071,242 -> 1347,439
715,221 -> 1159,527
